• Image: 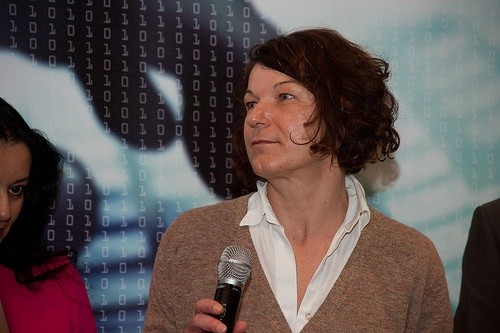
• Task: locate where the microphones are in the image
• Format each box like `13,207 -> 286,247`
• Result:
210,246 -> 252,333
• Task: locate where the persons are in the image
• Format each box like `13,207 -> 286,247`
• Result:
0,94 -> 103,333
452,196 -> 500,333
140,25 -> 457,333
